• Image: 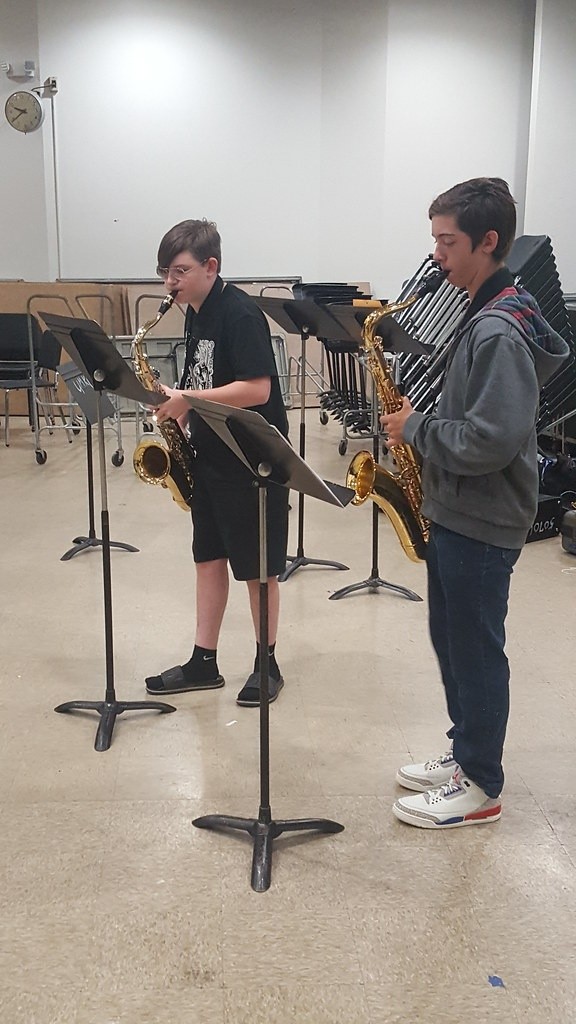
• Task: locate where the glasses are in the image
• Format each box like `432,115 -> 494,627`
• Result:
156,259 -> 209,281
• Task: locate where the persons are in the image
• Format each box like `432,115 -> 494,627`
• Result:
379,177 -> 569,828
143,218 -> 290,707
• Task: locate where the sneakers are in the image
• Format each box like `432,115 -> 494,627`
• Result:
396,745 -> 461,792
392,764 -> 503,829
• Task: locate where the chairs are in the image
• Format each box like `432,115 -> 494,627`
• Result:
0,313 -> 76,449
376,232 -> 576,460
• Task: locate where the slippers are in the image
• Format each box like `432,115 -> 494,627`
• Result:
236,671 -> 284,707
144,664 -> 225,695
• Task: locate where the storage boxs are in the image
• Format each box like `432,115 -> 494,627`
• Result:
526,494 -> 562,544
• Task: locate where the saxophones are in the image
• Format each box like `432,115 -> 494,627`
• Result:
130,290 -> 199,513
343,271 -> 451,565
